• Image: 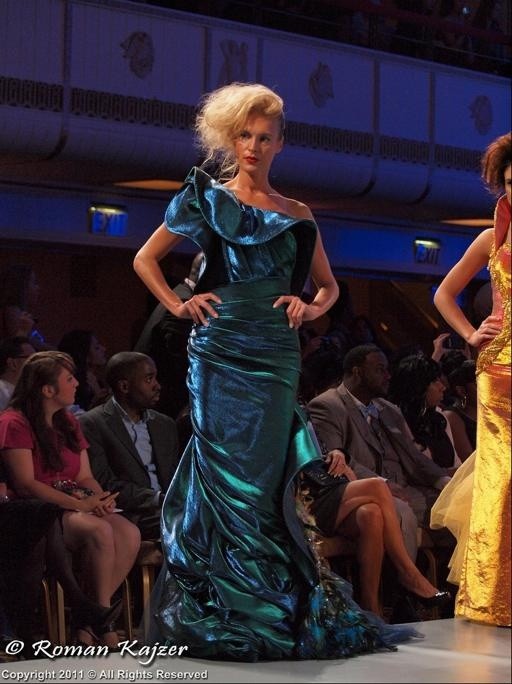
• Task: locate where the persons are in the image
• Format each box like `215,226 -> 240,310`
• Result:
0,308 -> 482,658
131,82 -> 424,664
428,130 -> 512,629
132,251 -> 203,419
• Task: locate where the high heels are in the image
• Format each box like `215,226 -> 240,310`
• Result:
71,599 -> 120,641
395,579 -> 451,611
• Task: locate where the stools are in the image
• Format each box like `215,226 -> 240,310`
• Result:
39,526 -> 455,656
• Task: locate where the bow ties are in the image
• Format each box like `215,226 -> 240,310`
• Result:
359,402 -> 378,419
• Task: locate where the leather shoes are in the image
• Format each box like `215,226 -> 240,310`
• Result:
75,626 -> 95,651
98,622 -> 121,652
